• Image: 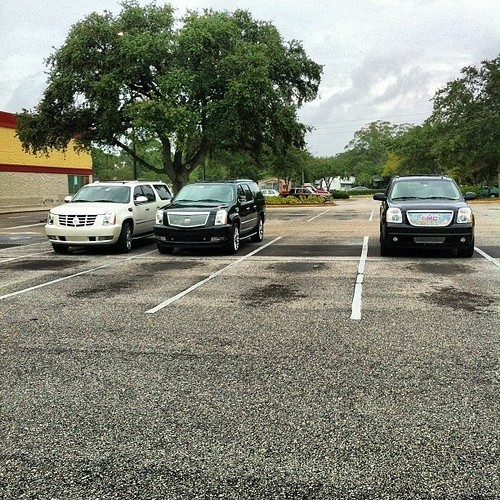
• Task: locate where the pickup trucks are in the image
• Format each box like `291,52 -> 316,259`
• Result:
281,185 -> 329,201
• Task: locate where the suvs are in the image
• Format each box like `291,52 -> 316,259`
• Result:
289,188 -> 326,203
152,178 -> 266,255
373,174 -> 475,258
44,180 -> 174,254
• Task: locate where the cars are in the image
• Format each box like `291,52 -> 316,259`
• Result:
350,187 -> 368,191
260,188 -> 280,198
